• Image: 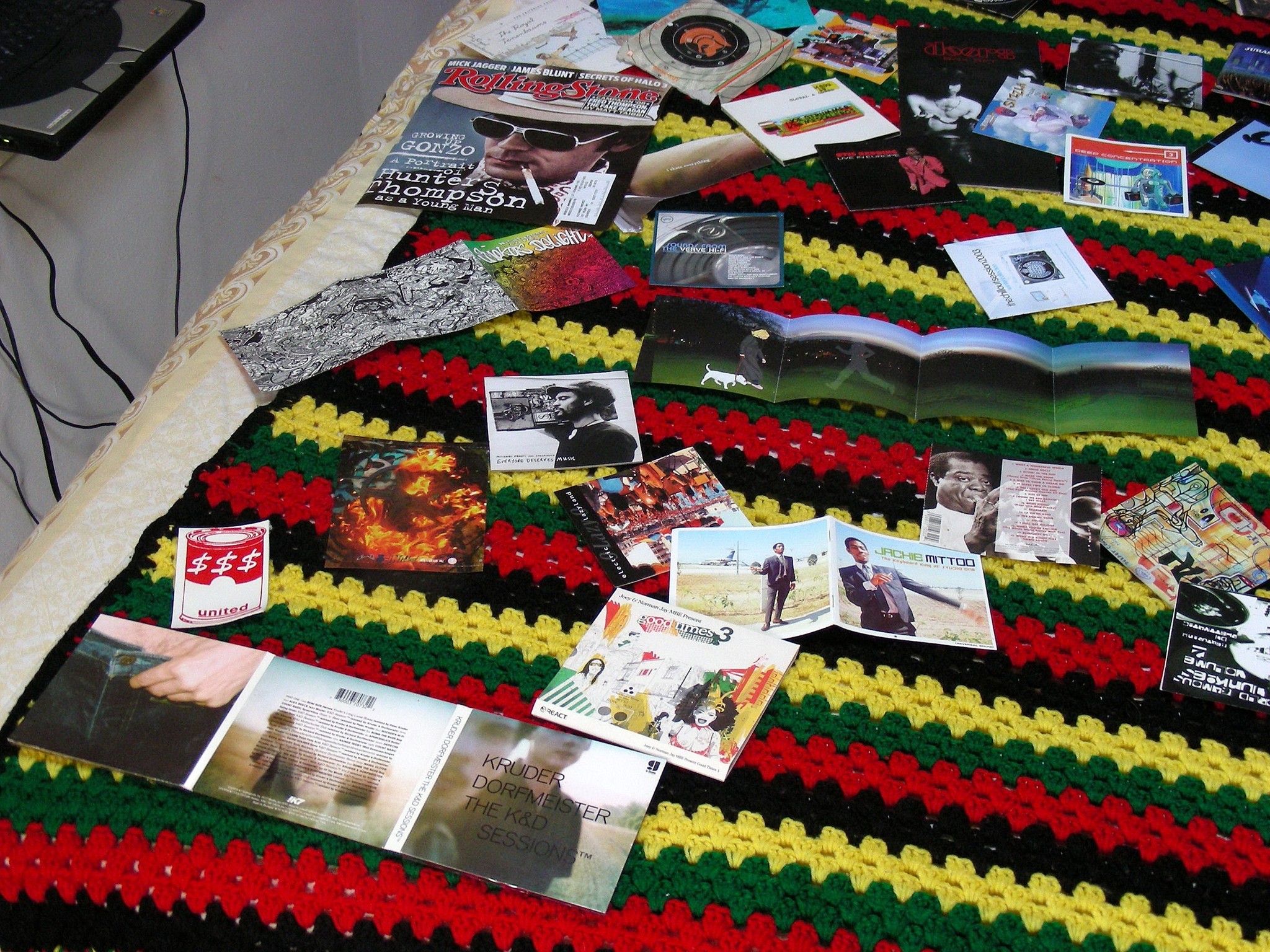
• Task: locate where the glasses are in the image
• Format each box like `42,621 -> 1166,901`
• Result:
590,662 -> 603,667
470,114 -> 618,153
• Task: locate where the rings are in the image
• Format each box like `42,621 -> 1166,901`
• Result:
985,499 -> 994,504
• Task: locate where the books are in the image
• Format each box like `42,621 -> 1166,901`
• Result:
170,434 -> 487,630
358,1 -> 1270,473
530,515 -> 997,782
919,442 -> 1270,712
6,612 -> 667,916
553,446 -> 754,588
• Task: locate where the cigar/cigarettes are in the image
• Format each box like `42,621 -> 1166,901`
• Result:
520,163 -> 545,205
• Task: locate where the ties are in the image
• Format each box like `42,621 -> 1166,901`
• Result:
781,555 -> 784,577
863,564 -> 890,613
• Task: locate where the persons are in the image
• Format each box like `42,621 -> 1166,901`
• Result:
1166,87 -> 1202,110
577,454 -> 719,532
921,451 -> 1077,565
1123,65 -> 1168,99
248,711 -> 318,815
750,543 -> 797,631
1067,39 -> 1138,94
898,146 -> 951,204
838,537 -> 986,637
353,113 -> 652,230
401,708 -> 591,894
9,613 -> 266,787
542,380 -> 637,468
907,70 -> 982,131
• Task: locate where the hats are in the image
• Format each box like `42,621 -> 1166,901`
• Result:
546,379 -> 615,413
433,85 -> 656,125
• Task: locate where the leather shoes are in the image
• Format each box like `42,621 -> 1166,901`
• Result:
772,619 -> 788,624
762,623 -> 770,631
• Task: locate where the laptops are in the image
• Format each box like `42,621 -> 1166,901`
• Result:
0,0 -> 206,160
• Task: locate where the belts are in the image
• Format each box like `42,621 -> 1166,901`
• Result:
882,614 -> 898,619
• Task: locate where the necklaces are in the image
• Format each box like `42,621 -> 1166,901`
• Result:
933,99 -> 960,112
568,419 -> 599,440
